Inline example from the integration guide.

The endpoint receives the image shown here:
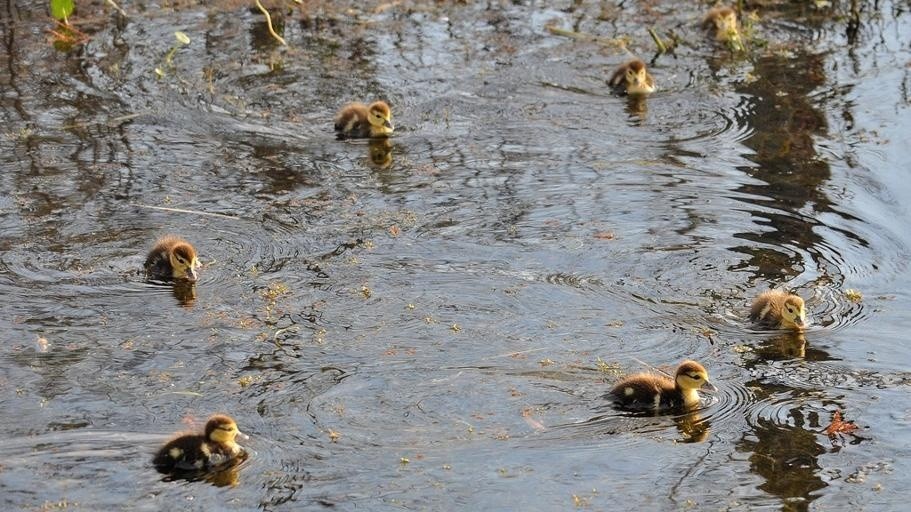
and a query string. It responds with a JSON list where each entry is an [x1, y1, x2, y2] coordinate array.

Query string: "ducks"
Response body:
[[609, 360, 719, 411], [143, 234, 203, 281], [367, 138, 396, 169], [672, 411, 708, 444], [750, 288, 806, 330], [700, 6, 737, 42], [334, 100, 395, 138], [152, 414, 250, 470], [608, 59, 655, 94], [780, 330, 807, 360]]
